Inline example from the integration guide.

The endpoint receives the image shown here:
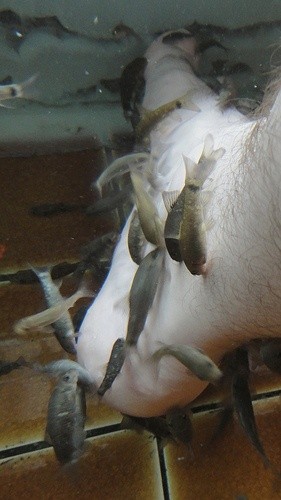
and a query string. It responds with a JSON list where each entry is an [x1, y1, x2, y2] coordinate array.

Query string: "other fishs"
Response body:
[[163, 154, 217, 276], [0, 355, 34, 376], [25, 259, 82, 354], [223, 346, 274, 469], [71, 384, 87, 459], [128, 208, 149, 265], [31, 203, 88, 217], [72, 304, 88, 333], [12, 269, 99, 336], [145, 340, 225, 386], [122, 408, 197, 460], [0, 76, 12, 85], [92, 338, 127, 404], [209, 406, 234, 451], [124, 246, 166, 346], [43, 368, 89, 483], [0, 72, 40, 111], [32, 358, 97, 394], [161, 134, 226, 262], [85, 183, 134, 218], [8, 232, 121, 287], [0, 10, 22, 26], [89, 21, 263, 245]]
